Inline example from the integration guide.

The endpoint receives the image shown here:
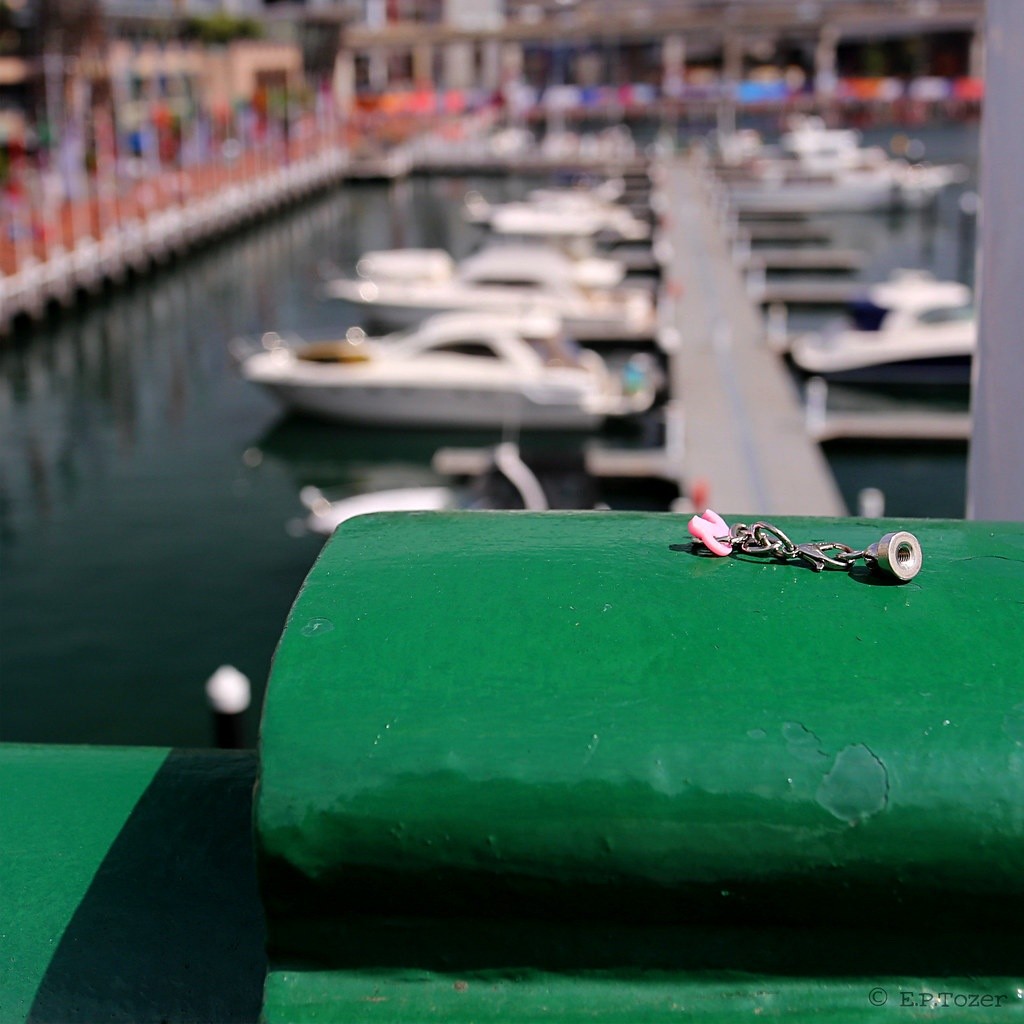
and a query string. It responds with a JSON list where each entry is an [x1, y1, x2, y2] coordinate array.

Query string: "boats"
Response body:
[[784, 273, 974, 379], [715, 113, 959, 217], [251, 162, 666, 534]]
